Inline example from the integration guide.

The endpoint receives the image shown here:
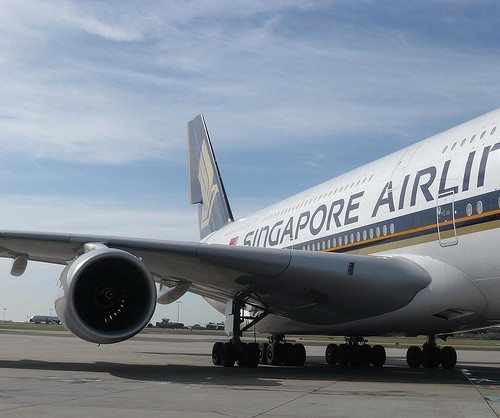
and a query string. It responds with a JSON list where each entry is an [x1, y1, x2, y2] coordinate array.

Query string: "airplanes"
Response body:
[[0, 107, 499, 372], [30, 315, 61, 325]]
